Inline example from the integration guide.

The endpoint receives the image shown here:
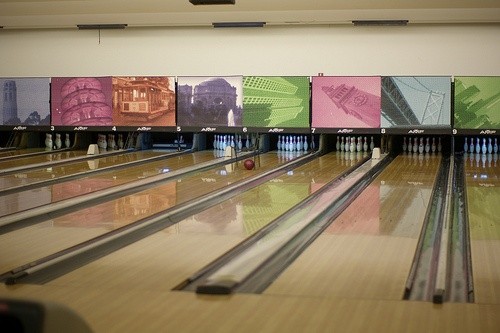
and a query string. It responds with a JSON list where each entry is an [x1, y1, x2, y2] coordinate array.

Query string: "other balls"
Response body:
[[244, 159, 255, 170]]
